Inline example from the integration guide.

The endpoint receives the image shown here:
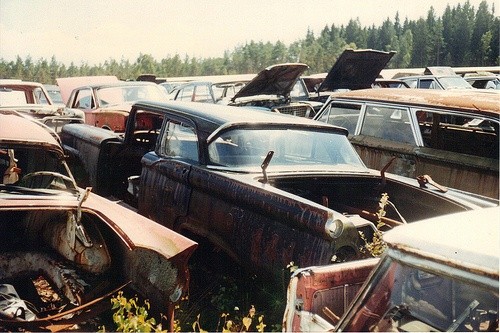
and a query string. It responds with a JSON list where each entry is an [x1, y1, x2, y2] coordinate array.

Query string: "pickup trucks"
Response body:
[[0, 0, 499, 333]]
